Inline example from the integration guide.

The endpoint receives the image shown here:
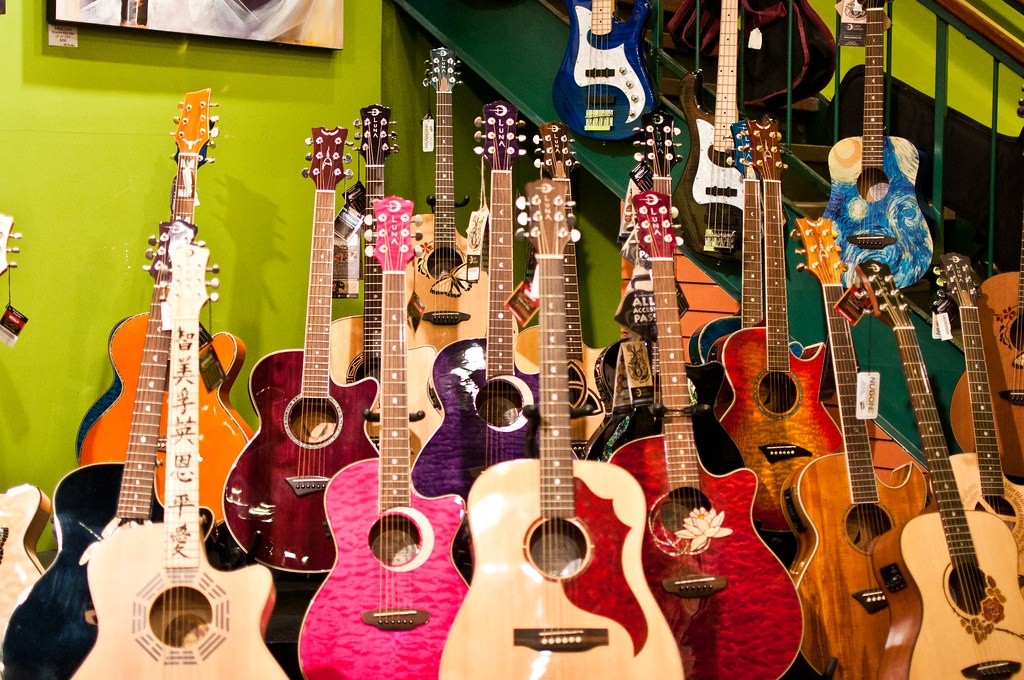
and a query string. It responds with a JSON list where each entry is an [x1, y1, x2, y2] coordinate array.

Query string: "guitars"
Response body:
[[852, 259, 1024, 680], [299, 196, 470, 680], [671, 0, 788, 263], [933, 249, 1024, 598], [785, 218, 929, 680], [333, 102, 414, 442], [75, 87, 254, 527], [410, 45, 490, 351], [220, 126, 382, 575], [72, 234, 291, 679], [821, 0, 934, 291], [515, 112, 846, 680], [0, 213, 54, 645], [410, 101, 541, 498], [551, 0, 659, 143], [0, 214, 219, 679], [949, 83, 1024, 481], [437, 176, 687, 679]]
